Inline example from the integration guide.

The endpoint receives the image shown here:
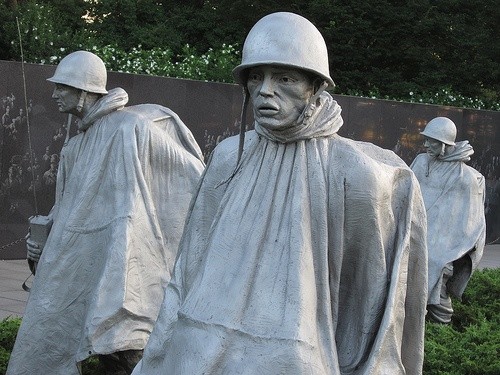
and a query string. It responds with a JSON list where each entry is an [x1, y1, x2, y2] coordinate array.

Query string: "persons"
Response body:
[[409, 117, 486, 323], [5, 50, 206, 375], [131, 12, 429, 375]]
